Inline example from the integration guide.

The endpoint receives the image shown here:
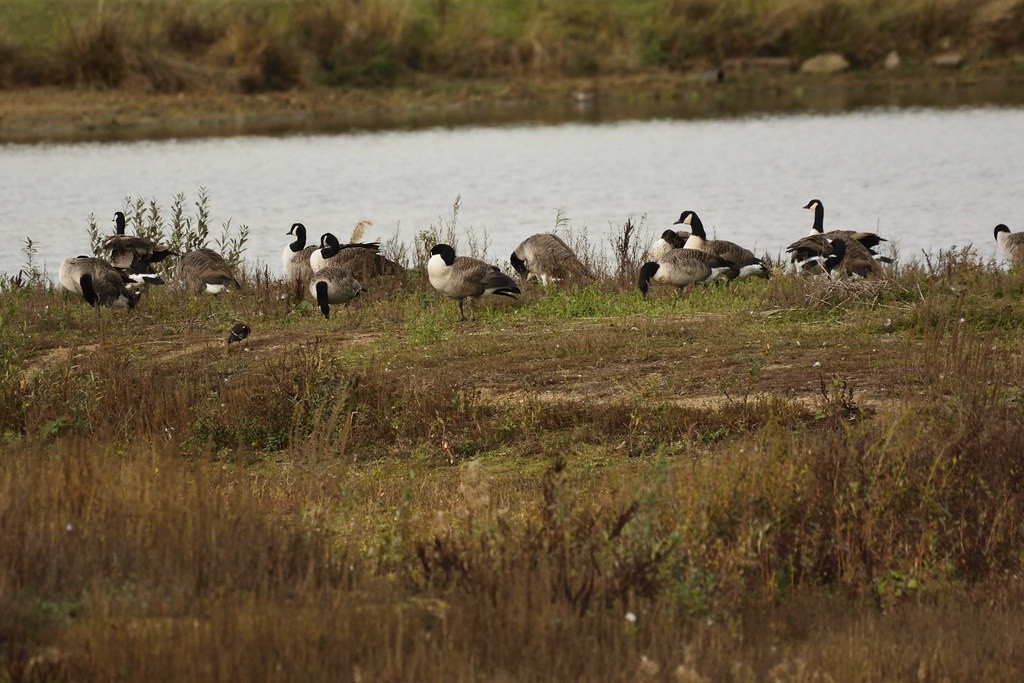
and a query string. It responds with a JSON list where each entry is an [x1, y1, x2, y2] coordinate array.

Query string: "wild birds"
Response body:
[[58, 255, 137, 307], [99, 210, 182, 286], [994, 224, 1024, 262], [176, 247, 242, 296], [282, 223, 382, 284], [310, 232, 406, 284], [784, 199, 894, 282], [226, 323, 252, 346], [426, 243, 522, 322], [636, 211, 772, 300], [510, 234, 596, 286], [309, 266, 369, 320]]
